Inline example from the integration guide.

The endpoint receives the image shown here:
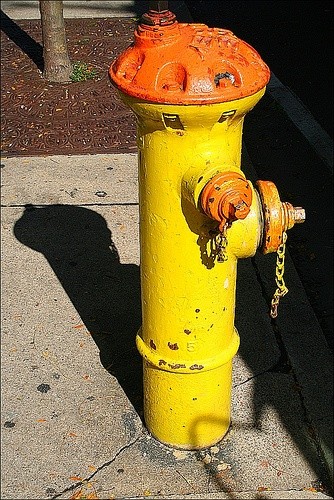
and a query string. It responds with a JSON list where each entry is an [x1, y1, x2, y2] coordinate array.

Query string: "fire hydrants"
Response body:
[[109, 6, 308, 453]]
